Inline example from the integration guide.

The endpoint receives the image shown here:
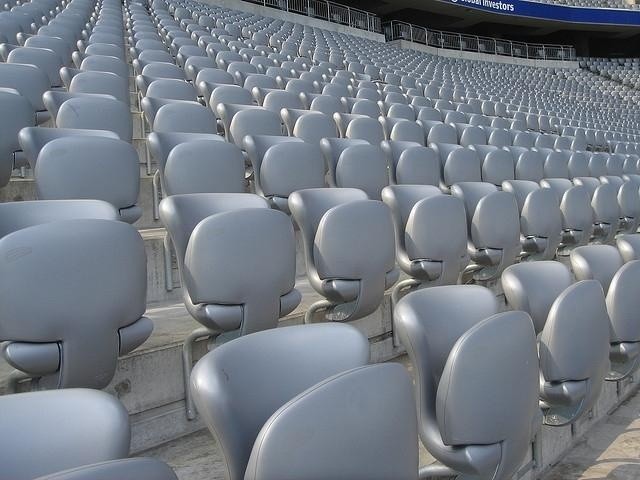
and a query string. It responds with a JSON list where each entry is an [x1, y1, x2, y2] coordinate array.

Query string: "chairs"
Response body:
[[1, 0, 640, 480]]
